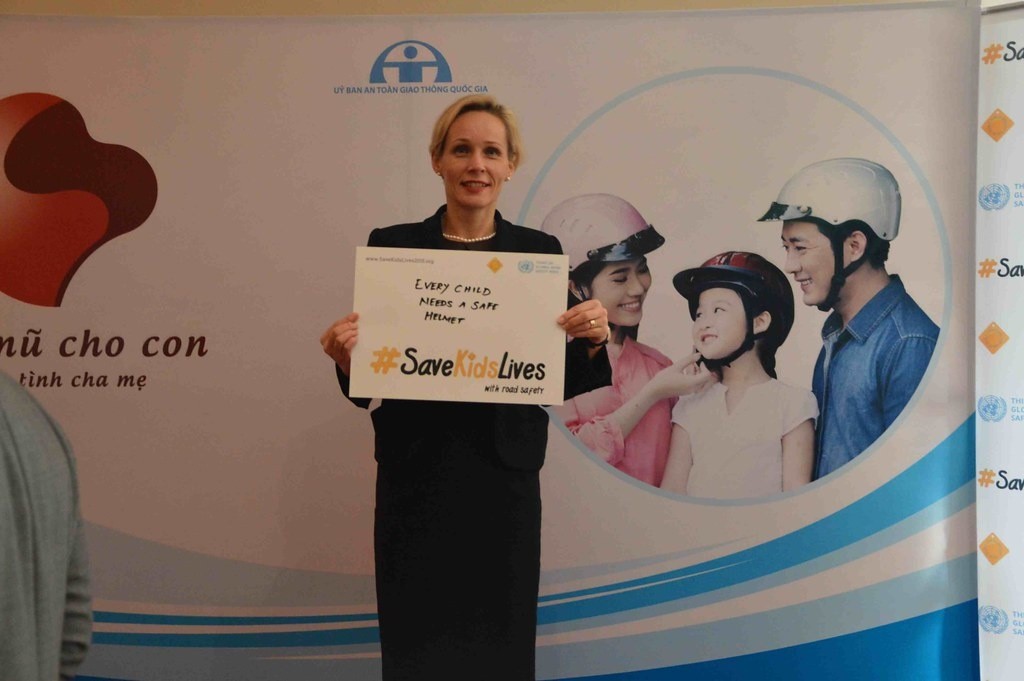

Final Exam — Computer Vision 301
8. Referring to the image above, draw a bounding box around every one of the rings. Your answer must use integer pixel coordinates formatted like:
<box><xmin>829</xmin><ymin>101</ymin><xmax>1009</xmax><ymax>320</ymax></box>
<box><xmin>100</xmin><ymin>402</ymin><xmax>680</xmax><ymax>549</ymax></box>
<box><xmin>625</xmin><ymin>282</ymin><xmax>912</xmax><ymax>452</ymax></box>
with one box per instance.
<box><xmin>590</xmin><ymin>319</ymin><xmax>596</xmax><ymax>327</ymax></box>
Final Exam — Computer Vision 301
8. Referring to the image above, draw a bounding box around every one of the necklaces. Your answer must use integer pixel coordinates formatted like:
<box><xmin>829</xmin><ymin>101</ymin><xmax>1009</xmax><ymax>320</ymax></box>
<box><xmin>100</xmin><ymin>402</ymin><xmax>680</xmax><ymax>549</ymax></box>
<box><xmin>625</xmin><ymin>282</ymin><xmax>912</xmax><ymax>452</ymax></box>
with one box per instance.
<box><xmin>443</xmin><ymin>231</ymin><xmax>496</xmax><ymax>242</ymax></box>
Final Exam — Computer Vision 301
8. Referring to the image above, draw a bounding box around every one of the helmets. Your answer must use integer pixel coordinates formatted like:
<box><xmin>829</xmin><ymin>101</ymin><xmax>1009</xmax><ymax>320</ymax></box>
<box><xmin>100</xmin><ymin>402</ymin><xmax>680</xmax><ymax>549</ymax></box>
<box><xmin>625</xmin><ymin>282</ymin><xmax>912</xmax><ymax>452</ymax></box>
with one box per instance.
<box><xmin>672</xmin><ymin>251</ymin><xmax>795</xmax><ymax>345</ymax></box>
<box><xmin>757</xmin><ymin>158</ymin><xmax>901</xmax><ymax>242</ymax></box>
<box><xmin>540</xmin><ymin>194</ymin><xmax>665</xmax><ymax>287</ymax></box>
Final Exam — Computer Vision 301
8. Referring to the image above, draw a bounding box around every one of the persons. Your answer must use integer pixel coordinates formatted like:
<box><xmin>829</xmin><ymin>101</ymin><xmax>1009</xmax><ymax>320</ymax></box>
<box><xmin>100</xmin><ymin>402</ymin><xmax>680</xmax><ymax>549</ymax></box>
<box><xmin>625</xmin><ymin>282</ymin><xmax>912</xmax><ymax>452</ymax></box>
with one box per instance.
<box><xmin>318</xmin><ymin>93</ymin><xmax>609</xmax><ymax>681</ymax></box>
<box><xmin>540</xmin><ymin>193</ymin><xmax>711</xmax><ymax>488</ymax></box>
<box><xmin>755</xmin><ymin>158</ymin><xmax>940</xmax><ymax>483</ymax></box>
<box><xmin>659</xmin><ymin>252</ymin><xmax>819</xmax><ymax>499</ymax></box>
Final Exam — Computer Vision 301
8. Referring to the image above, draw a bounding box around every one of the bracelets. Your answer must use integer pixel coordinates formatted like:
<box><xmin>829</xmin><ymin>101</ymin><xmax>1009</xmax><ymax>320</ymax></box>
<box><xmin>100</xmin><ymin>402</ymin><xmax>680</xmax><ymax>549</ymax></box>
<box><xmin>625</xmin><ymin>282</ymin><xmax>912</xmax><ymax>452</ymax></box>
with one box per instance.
<box><xmin>587</xmin><ymin>333</ymin><xmax>608</xmax><ymax>346</ymax></box>
